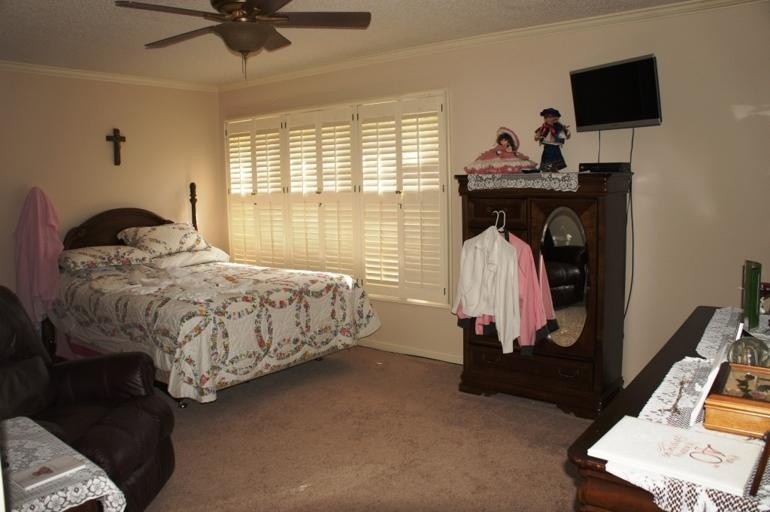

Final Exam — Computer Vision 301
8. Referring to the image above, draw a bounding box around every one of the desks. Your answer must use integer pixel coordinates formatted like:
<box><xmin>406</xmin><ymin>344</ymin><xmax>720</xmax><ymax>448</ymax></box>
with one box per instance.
<box><xmin>567</xmin><ymin>306</ymin><xmax>770</xmax><ymax>512</ymax></box>
<box><xmin>0</xmin><ymin>416</ymin><xmax>127</xmax><ymax>512</ymax></box>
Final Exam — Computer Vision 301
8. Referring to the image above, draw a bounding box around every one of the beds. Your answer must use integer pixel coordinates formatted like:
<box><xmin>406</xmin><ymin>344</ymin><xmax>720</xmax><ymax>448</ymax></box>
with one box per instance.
<box><xmin>34</xmin><ymin>182</ymin><xmax>381</xmax><ymax>408</ymax></box>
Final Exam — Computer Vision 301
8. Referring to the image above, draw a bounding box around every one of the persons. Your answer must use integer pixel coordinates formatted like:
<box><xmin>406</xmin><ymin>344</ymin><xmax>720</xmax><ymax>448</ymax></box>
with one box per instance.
<box><xmin>533</xmin><ymin>107</ymin><xmax>571</xmax><ymax>174</ymax></box>
<box><xmin>464</xmin><ymin>127</ymin><xmax>538</xmax><ymax>174</ymax></box>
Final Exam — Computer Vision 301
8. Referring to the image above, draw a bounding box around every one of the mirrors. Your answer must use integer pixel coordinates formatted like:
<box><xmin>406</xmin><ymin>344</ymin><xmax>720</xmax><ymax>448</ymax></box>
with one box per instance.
<box><xmin>540</xmin><ymin>207</ymin><xmax>589</xmax><ymax>347</ymax></box>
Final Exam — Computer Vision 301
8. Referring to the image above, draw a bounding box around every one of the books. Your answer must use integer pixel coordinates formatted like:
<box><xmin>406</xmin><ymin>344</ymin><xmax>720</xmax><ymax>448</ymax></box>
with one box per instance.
<box><xmin>9</xmin><ymin>455</ymin><xmax>86</xmax><ymax>491</ymax></box>
<box><xmin>586</xmin><ymin>413</ymin><xmax>770</xmax><ymax>497</ymax></box>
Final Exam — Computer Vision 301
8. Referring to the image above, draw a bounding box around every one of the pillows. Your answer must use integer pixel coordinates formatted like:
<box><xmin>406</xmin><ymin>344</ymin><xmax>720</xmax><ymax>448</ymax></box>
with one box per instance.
<box><xmin>58</xmin><ymin>246</ymin><xmax>152</xmax><ymax>272</ymax></box>
<box><xmin>118</xmin><ymin>223</ymin><xmax>210</xmax><ymax>259</ymax></box>
<box><xmin>149</xmin><ymin>247</ymin><xmax>231</xmax><ymax>269</ymax></box>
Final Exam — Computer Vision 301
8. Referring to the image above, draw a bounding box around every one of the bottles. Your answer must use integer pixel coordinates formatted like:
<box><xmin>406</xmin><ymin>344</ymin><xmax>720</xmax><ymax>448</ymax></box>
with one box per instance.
<box><xmin>726</xmin><ymin>337</ymin><xmax>770</xmax><ymax>371</ymax></box>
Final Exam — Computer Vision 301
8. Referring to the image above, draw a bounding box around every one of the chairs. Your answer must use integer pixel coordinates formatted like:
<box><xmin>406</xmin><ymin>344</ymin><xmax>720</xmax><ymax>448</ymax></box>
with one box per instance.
<box><xmin>0</xmin><ymin>285</ymin><xmax>174</xmax><ymax>512</ymax></box>
<box><xmin>545</xmin><ymin>229</ymin><xmax>585</xmax><ymax>308</ymax></box>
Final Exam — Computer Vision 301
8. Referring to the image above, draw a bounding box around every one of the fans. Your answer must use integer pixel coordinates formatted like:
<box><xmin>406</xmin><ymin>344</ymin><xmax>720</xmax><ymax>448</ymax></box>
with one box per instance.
<box><xmin>114</xmin><ymin>0</ymin><xmax>370</xmax><ymax>51</ymax></box>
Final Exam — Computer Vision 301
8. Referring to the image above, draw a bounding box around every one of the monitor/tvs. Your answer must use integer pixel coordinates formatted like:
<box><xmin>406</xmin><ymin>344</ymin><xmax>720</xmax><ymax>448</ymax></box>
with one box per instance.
<box><xmin>570</xmin><ymin>54</ymin><xmax>662</xmax><ymax>132</ymax></box>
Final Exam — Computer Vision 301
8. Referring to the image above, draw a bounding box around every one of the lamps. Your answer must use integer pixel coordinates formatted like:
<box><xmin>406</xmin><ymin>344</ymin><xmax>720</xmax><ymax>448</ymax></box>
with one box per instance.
<box><xmin>215</xmin><ymin>22</ymin><xmax>268</xmax><ymax>79</ymax></box>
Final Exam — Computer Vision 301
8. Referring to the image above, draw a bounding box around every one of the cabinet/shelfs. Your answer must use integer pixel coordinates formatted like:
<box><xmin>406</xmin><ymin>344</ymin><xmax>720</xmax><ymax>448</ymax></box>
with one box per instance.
<box><xmin>455</xmin><ymin>171</ymin><xmax>635</xmax><ymax>420</ymax></box>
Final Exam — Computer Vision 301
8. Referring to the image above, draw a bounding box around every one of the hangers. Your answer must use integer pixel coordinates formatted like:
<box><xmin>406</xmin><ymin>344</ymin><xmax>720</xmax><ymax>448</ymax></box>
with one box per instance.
<box><xmin>492</xmin><ymin>211</ymin><xmax>506</xmax><ymax>232</ymax></box>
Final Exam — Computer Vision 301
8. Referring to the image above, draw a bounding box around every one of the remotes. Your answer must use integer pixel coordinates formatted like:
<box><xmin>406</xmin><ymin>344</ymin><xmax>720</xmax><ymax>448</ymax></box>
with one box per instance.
<box><xmin>521</xmin><ymin>167</ymin><xmax>540</xmax><ymax>173</ymax></box>
